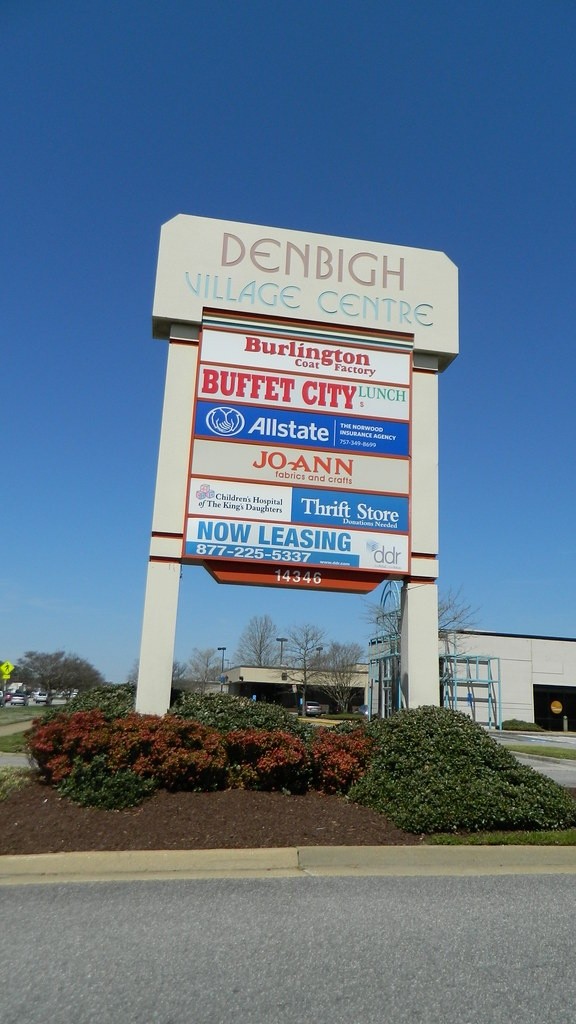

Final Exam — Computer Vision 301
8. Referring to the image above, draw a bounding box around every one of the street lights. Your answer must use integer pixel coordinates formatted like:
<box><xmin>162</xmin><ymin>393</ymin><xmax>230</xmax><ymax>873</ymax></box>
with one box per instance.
<box><xmin>316</xmin><ymin>646</ymin><xmax>323</xmax><ymax>661</ymax></box>
<box><xmin>218</xmin><ymin>647</ymin><xmax>227</xmax><ymax>672</ymax></box>
<box><xmin>276</xmin><ymin>637</ymin><xmax>288</xmax><ymax>665</ymax></box>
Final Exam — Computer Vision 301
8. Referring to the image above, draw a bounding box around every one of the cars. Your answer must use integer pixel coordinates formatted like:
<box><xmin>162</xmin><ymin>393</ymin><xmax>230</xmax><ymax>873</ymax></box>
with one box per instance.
<box><xmin>0</xmin><ymin>687</ymin><xmax>79</xmax><ymax>708</ymax></box>
<box><xmin>297</xmin><ymin>700</ymin><xmax>322</xmax><ymax>718</ymax></box>
